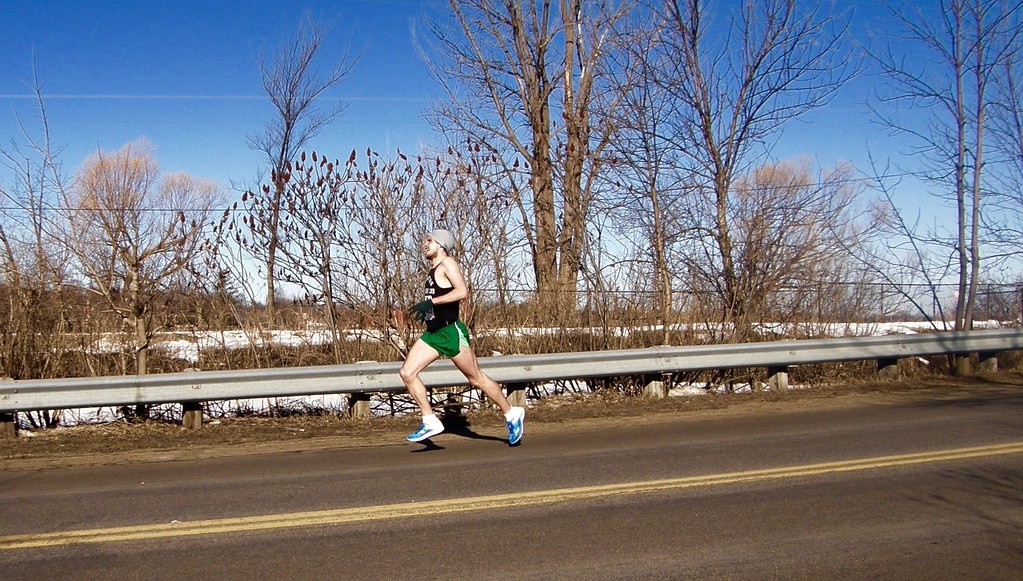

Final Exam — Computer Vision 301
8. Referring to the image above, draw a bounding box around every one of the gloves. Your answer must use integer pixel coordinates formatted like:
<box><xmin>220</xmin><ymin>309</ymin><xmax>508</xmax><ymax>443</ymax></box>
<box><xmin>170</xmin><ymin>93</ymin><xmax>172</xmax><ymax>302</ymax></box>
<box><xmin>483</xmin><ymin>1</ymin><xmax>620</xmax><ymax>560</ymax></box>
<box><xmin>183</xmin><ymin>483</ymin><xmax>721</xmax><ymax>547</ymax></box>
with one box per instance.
<box><xmin>407</xmin><ymin>299</ymin><xmax>434</xmax><ymax>325</ymax></box>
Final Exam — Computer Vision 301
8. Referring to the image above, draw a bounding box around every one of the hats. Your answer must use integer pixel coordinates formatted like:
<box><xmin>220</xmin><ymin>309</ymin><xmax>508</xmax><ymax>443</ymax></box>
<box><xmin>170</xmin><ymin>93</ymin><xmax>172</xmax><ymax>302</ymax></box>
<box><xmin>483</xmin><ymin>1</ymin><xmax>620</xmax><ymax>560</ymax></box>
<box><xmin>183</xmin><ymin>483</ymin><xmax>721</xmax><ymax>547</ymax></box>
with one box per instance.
<box><xmin>428</xmin><ymin>229</ymin><xmax>455</xmax><ymax>254</ymax></box>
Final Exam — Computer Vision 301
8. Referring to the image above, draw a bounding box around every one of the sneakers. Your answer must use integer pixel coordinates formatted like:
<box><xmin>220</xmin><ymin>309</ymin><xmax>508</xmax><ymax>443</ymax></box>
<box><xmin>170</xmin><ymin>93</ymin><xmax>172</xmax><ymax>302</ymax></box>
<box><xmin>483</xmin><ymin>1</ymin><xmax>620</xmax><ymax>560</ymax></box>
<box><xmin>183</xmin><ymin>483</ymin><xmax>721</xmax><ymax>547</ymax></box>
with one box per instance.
<box><xmin>407</xmin><ymin>423</ymin><xmax>445</xmax><ymax>441</ymax></box>
<box><xmin>503</xmin><ymin>407</ymin><xmax>525</xmax><ymax>444</ymax></box>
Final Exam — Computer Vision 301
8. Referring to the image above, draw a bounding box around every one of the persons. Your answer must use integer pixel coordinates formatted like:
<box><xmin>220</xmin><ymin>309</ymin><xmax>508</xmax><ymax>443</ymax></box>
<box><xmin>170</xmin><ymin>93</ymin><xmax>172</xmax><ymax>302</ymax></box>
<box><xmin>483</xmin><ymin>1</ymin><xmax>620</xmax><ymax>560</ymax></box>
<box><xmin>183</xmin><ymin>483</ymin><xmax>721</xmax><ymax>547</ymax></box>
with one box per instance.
<box><xmin>398</xmin><ymin>229</ymin><xmax>525</xmax><ymax>444</ymax></box>
